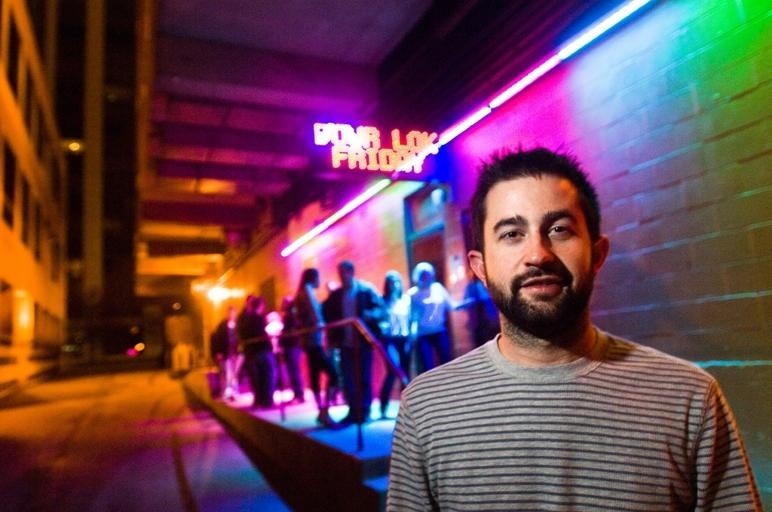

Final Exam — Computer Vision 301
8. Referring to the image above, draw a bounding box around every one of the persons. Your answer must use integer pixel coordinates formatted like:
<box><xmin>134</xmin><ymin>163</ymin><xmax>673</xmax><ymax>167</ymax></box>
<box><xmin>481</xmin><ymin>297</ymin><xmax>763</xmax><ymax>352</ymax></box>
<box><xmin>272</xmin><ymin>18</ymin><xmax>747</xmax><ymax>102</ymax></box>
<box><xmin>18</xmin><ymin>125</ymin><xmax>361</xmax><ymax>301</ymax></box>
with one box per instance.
<box><xmin>209</xmin><ymin>293</ymin><xmax>305</xmax><ymax>408</ymax></box>
<box><xmin>293</xmin><ymin>260</ymin><xmax>453</xmax><ymax>432</ymax></box>
<box><xmin>453</xmin><ymin>278</ymin><xmax>500</xmax><ymax>349</ymax></box>
<box><xmin>385</xmin><ymin>147</ymin><xmax>764</xmax><ymax>512</ymax></box>
